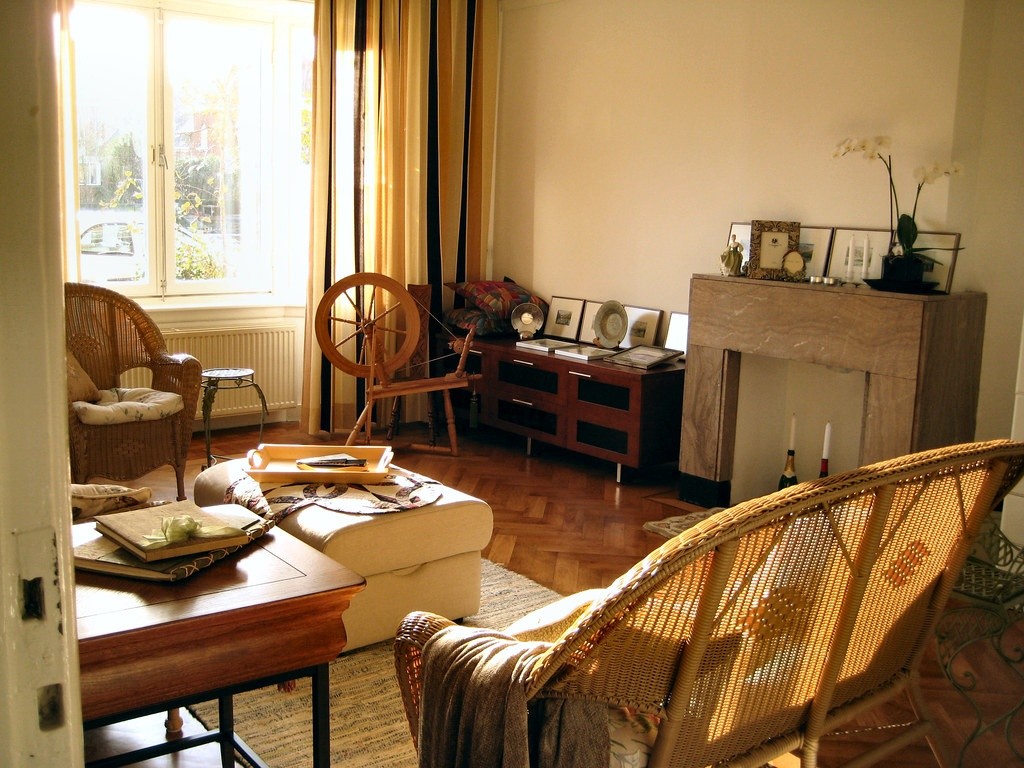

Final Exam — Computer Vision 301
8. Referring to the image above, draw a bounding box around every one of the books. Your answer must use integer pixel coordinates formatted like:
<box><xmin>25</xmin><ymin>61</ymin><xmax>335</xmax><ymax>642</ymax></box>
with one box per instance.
<box><xmin>74</xmin><ymin>500</ymin><xmax>276</xmax><ymax>581</ymax></box>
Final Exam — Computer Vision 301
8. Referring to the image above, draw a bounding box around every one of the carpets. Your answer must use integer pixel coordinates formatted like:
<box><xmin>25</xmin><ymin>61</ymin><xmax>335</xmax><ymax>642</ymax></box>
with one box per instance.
<box><xmin>642</xmin><ymin>507</ymin><xmax>726</xmax><ymax>539</ymax></box>
<box><xmin>190</xmin><ymin>554</ymin><xmax>563</xmax><ymax>768</ymax></box>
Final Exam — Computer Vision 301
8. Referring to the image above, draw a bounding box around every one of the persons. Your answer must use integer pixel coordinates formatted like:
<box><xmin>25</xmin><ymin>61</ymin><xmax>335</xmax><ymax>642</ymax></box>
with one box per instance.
<box><xmin>727</xmin><ymin>235</ymin><xmax>743</xmax><ymax>274</ymax></box>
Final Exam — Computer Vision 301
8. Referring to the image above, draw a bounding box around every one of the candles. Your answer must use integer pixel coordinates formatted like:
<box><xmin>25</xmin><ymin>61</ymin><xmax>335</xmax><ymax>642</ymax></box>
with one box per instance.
<box><xmin>821</xmin><ymin>419</ymin><xmax>831</xmax><ymax>459</ymax></box>
<box><xmin>848</xmin><ymin>233</ymin><xmax>856</xmax><ymax>270</ymax></box>
<box><xmin>863</xmin><ymin>233</ymin><xmax>870</xmax><ymax>273</ymax></box>
<box><xmin>789</xmin><ymin>411</ymin><xmax>797</xmax><ymax>450</ymax></box>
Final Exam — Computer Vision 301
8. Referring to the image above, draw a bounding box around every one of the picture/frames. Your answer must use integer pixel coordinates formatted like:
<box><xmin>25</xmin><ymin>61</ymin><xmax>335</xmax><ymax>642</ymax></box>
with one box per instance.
<box><xmin>603</xmin><ymin>342</ymin><xmax>685</xmax><ymax>370</ymax></box>
<box><xmin>892</xmin><ymin>231</ymin><xmax>962</xmax><ymax>296</ymax></box>
<box><xmin>577</xmin><ymin>300</ymin><xmax>625</xmax><ymax>349</ymax></box>
<box><xmin>662</xmin><ymin>310</ymin><xmax>688</xmax><ymax>359</ymax></box>
<box><xmin>826</xmin><ymin>227</ymin><xmax>894</xmax><ymax>285</ymax></box>
<box><xmin>747</xmin><ymin>219</ymin><xmax>800</xmax><ymax>282</ymax></box>
<box><xmin>799</xmin><ymin>224</ymin><xmax>834</xmax><ymax>282</ymax></box>
<box><xmin>618</xmin><ymin>304</ymin><xmax>665</xmax><ymax>349</ymax></box>
<box><xmin>726</xmin><ymin>221</ymin><xmax>751</xmax><ymax>275</ymax></box>
<box><xmin>542</xmin><ymin>294</ymin><xmax>586</xmax><ymax>343</ymax></box>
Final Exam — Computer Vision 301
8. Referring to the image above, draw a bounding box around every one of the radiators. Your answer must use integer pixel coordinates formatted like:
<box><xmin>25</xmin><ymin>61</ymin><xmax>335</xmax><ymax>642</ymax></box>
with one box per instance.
<box><xmin>120</xmin><ymin>322</ymin><xmax>304</xmax><ymax>420</ymax></box>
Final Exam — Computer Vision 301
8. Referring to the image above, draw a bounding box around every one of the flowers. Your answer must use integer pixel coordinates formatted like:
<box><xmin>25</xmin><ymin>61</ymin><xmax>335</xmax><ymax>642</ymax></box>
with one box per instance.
<box><xmin>831</xmin><ymin>134</ymin><xmax>965</xmax><ymax>267</ymax></box>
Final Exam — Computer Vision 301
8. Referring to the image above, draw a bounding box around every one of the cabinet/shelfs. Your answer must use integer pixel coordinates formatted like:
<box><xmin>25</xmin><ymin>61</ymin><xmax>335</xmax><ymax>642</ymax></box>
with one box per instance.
<box><xmin>449</xmin><ymin>335</ymin><xmax>689</xmax><ymax>486</ymax></box>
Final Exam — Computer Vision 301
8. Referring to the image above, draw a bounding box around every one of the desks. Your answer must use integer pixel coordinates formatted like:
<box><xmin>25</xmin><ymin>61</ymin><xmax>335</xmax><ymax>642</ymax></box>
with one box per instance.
<box><xmin>932</xmin><ymin>514</ymin><xmax>1024</xmax><ymax>768</ymax></box>
<box><xmin>72</xmin><ymin>503</ymin><xmax>368</xmax><ymax>768</ymax></box>
<box><xmin>200</xmin><ymin>367</ymin><xmax>270</xmax><ymax>472</ymax></box>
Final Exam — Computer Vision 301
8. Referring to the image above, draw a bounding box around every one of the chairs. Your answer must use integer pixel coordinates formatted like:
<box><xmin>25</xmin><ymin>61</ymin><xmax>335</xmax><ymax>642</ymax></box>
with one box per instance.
<box><xmin>65</xmin><ymin>281</ymin><xmax>216</xmax><ymax>499</ymax></box>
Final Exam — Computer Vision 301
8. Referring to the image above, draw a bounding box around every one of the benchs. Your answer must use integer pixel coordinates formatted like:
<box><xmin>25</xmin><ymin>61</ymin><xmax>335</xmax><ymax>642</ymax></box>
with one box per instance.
<box><xmin>193</xmin><ymin>458</ymin><xmax>494</xmax><ymax>647</ymax></box>
<box><xmin>393</xmin><ymin>438</ymin><xmax>1024</xmax><ymax>768</ymax></box>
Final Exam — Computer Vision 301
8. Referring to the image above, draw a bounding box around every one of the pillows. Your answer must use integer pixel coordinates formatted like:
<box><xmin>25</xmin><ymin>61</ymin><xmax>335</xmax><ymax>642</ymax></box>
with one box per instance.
<box><xmin>71</xmin><ymin>482</ymin><xmax>153</xmax><ymax>520</ymax></box>
<box><xmin>66</xmin><ymin>349</ymin><xmax>102</xmax><ymax>401</ymax></box>
<box><xmin>442</xmin><ymin>274</ymin><xmax>550</xmax><ymax>321</ymax></box>
<box><xmin>442</xmin><ymin>307</ymin><xmax>520</xmax><ymax>339</ymax></box>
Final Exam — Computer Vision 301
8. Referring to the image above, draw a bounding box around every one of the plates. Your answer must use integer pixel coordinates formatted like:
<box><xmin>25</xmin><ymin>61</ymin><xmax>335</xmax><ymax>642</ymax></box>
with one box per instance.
<box><xmin>511</xmin><ymin>303</ymin><xmax>544</xmax><ymax>334</ymax></box>
<box><xmin>594</xmin><ymin>300</ymin><xmax>628</xmax><ymax>349</ymax></box>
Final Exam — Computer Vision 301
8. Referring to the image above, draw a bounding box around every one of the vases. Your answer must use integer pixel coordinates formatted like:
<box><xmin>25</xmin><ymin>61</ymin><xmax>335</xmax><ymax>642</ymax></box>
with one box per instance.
<box><xmin>863</xmin><ymin>255</ymin><xmax>940</xmax><ymax>294</ymax></box>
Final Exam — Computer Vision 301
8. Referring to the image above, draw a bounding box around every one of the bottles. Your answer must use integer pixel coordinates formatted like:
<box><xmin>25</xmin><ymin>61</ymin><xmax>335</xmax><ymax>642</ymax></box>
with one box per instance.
<box><xmin>777</xmin><ymin>449</ymin><xmax>798</xmax><ymax>491</ymax></box>
<box><xmin>819</xmin><ymin>458</ymin><xmax>829</xmax><ymax>478</ymax></box>
